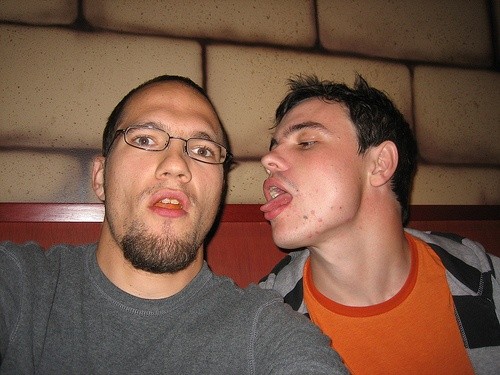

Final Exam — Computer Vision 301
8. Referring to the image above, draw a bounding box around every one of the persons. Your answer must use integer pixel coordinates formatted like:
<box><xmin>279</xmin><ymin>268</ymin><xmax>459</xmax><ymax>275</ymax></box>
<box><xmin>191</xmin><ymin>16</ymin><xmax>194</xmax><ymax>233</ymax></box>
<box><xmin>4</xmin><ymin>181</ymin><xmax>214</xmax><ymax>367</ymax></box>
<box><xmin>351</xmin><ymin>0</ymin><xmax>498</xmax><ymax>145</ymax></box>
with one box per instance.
<box><xmin>257</xmin><ymin>71</ymin><xmax>500</xmax><ymax>374</ymax></box>
<box><xmin>1</xmin><ymin>74</ymin><xmax>350</xmax><ymax>374</ymax></box>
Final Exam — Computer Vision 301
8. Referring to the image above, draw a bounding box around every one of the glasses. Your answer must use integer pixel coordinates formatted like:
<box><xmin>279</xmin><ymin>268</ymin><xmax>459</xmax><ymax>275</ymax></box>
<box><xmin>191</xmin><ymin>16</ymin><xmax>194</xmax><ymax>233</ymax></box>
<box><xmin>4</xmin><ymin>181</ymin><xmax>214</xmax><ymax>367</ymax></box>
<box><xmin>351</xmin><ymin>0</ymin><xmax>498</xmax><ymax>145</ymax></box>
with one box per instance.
<box><xmin>108</xmin><ymin>124</ymin><xmax>234</xmax><ymax>165</ymax></box>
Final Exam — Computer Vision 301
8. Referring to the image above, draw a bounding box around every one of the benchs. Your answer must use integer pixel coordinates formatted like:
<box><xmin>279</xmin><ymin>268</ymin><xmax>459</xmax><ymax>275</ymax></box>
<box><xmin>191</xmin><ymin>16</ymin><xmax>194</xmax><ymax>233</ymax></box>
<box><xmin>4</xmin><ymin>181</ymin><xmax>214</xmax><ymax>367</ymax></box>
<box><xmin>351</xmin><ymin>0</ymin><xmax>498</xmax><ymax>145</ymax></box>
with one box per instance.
<box><xmin>0</xmin><ymin>203</ymin><xmax>500</xmax><ymax>290</ymax></box>
